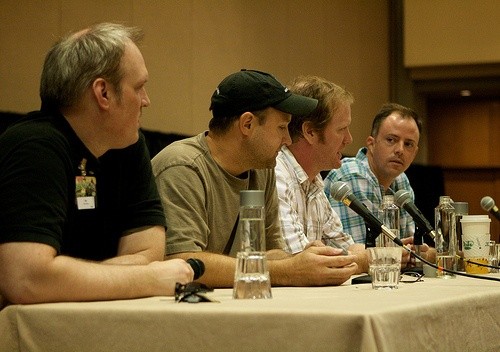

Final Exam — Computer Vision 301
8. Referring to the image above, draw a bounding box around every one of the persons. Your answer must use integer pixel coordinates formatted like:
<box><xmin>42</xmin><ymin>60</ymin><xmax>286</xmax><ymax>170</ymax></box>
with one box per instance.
<box><xmin>0</xmin><ymin>22</ymin><xmax>194</xmax><ymax>311</ymax></box>
<box><xmin>274</xmin><ymin>75</ymin><xmax>429</xmax><ymax>275</ymax></box>
<box><xmin>325</xmin><ymin>103</ymin><xmax>464</xmax><ymax>269</ymax></box>
<box><xmin>151</xmin><ymin>68</ymin><xmax>358</xmax><ymax>287</ymax></box>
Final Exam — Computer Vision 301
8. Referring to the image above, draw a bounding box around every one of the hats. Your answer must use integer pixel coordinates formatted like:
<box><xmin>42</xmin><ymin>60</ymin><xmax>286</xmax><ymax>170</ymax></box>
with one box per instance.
<box><xmin>212</xmin><ymin>68</ymin><xmax>319</xmax><ymax>116</ymax></box>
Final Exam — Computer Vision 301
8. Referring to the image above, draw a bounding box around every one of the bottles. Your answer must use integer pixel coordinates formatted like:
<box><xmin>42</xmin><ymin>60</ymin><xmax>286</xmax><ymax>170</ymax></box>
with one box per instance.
<box><xmin>434</xmin><ymin>196</ymin><xmax>457</xmax><ymax>280</ymax></box>
<box><xmin>378</xmin><ymin>194</ymin><xmax>400</xmax><ymax>247</ymax></box>
<box><xmin>239</xmin><ymin>190</ymin><xmax>267</xmax><ymax>254</ymax></box>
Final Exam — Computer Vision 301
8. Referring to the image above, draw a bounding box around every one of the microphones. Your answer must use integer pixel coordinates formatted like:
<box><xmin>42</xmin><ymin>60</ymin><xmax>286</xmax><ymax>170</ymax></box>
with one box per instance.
<box><xmin>393</xmin><ymin>189</ymin><xmax>437</xmax><ymax>241</ymax></box>
<box><xmin>329</xmin><ymin>181</ymin><xmax>404</xmax><ymax>246</ymax></box>
<box><xmin>480</xmin><ymin>195</ymin><xmax>500</xmax><ymax>222</ymax></box>
<box><xmin>185</xmin><ymin>257</ymin><xmax>206</xmax><ymax>280</ymax></box>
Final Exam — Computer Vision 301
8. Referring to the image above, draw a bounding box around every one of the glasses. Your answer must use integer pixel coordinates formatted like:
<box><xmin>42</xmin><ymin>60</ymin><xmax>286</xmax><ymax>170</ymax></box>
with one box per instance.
<box><xmin>400</xmin><ymin>271</ymin><xmax>425</xmax><ymax>283</ymax></box>
<box><xmin>174</xmin><ymin>281</ymin><xmax>215</xmax><ymax>303</ymax></box>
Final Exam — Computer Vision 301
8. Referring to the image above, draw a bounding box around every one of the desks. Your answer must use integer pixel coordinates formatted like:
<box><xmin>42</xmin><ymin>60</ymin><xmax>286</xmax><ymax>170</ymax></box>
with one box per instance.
<box><xmin>0</xmin><ymin>268</ymin><xmax>500</xmax><ymax>352</ymax></box>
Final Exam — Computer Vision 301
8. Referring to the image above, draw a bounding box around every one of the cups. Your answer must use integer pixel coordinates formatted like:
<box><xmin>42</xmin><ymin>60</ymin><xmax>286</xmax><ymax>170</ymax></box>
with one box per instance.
<box><xmin>489</xmin><ymin>244</ymin><xmax>500</xmax><ymax>273</ymax></box>
<box><xmin>460</xmin><ymin>214</ymin><xmax>492</xmax><ymax>275</ymax></box>
<box><xmin>365</xmin><ymin>245</ymin><xmax>402</xmax><ymax>290</ymax></box>
<box><xmin>232</xmin><ymin>250</ymin><xmax>273</xmax><ymax>299</ymax></box>
